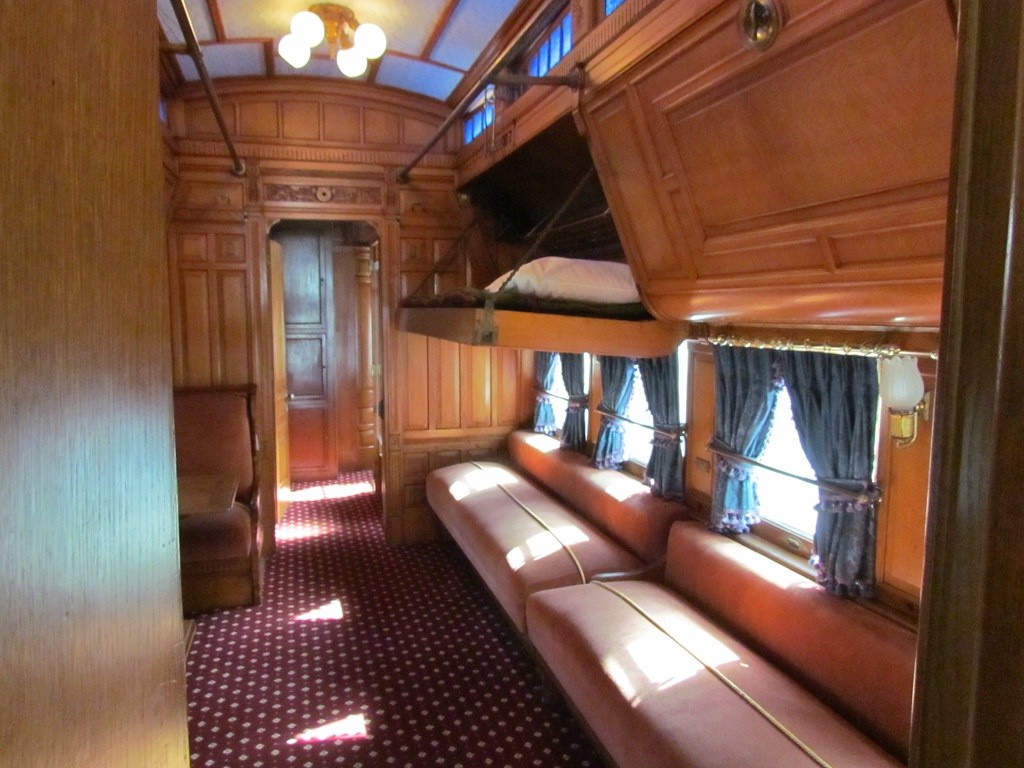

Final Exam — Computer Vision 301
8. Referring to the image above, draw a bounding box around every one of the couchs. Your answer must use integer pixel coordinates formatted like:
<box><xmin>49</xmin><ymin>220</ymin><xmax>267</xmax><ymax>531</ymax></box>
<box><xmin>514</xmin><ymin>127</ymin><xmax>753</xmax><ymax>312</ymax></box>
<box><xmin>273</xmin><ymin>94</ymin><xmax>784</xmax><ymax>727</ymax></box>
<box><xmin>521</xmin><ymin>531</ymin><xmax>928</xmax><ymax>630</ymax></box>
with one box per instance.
<box><xmin>173</xmin><ymin>383</ymin><xmax>266</xmax><ymax>611</ymax></box>
<box><xmin>426</xmin><ymin>431</ymin><xmax>685</xmax><ymax>634</ymax></box>
<box><xmin>525</xmin><ymin>521</ymin><xmax>919</xmax><ymax>768</ymax></box>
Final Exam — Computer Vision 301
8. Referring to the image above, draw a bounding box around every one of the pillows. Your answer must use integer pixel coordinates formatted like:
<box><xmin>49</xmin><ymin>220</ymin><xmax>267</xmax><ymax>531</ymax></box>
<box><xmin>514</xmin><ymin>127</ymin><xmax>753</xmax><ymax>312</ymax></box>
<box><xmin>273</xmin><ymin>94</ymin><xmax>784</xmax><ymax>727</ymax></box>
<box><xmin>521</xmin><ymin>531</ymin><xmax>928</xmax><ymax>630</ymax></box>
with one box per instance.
<box><xmin>484</xmin><ymin>256</ymin><xmax>639</xmax><ymax>304</ymax></box>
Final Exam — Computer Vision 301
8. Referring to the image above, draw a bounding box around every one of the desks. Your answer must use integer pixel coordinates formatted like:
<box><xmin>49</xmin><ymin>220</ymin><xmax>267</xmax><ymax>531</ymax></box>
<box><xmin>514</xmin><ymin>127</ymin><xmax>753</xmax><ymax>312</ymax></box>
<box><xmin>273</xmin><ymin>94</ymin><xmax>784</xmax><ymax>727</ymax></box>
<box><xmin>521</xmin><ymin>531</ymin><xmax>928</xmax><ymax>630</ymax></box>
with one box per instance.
<box><xmin>178</xmin><ymin>472</ymin><xmax>240</xmax><ymax>517</ymax></box>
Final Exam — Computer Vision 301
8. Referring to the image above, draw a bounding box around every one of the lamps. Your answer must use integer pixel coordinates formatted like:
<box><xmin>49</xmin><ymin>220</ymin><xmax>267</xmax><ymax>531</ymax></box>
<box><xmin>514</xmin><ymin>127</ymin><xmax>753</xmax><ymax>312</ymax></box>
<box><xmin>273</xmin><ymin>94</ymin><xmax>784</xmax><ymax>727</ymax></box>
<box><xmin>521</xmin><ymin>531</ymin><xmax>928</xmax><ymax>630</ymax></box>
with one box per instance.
<box><xmin>278</xmin><ymin>3</ymin><xmax>386</xmax><ymax>78</ymax></box>
<box><xmin>877</xmin><ymin>356</ymin><xmax>932</xmax><ymax>449</ymax></box>
